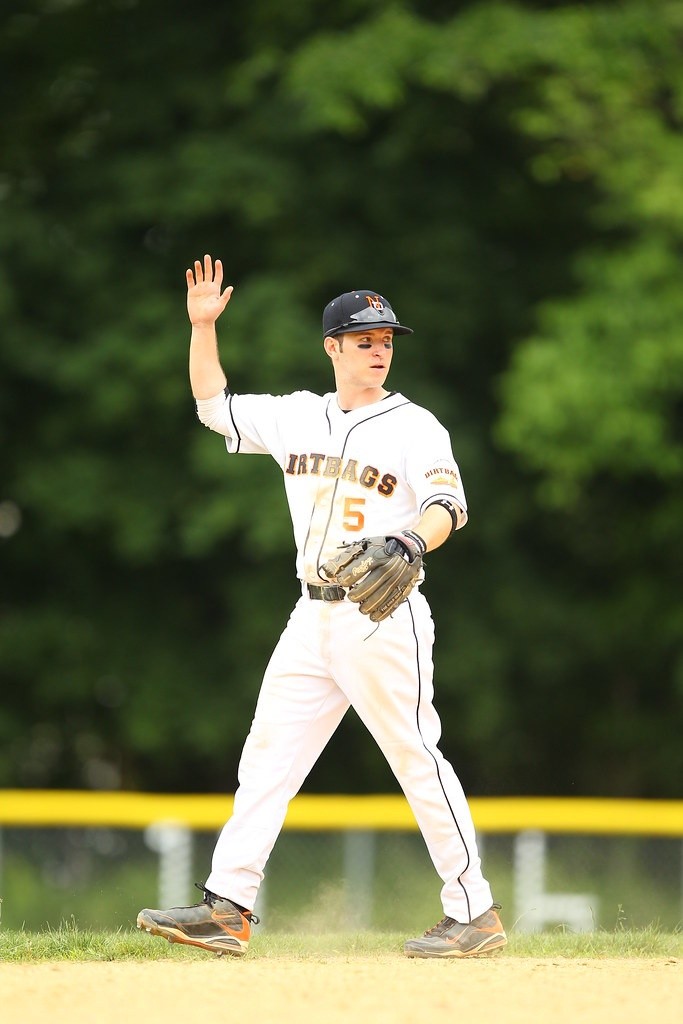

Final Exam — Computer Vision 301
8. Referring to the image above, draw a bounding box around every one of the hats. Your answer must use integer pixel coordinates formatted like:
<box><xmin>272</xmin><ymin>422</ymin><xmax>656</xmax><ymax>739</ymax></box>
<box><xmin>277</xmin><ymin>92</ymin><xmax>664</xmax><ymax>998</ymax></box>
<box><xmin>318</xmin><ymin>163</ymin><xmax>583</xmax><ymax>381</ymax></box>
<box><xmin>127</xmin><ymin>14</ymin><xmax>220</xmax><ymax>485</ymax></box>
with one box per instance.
<box><xmin>322</xmin><ymin>290</ymin><xmax>413</xmax><ymax>341</ymax></box>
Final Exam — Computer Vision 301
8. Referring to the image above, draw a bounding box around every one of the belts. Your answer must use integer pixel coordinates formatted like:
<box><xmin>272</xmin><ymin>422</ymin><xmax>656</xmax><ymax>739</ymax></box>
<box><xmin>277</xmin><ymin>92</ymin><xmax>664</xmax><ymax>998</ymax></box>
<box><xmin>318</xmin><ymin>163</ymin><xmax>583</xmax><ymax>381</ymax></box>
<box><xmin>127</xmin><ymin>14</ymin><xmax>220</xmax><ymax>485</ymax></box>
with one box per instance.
<box><xmin>300</xmin><ymin>580</ymin><xmax>349</xmax><ymax>603</ymax></box>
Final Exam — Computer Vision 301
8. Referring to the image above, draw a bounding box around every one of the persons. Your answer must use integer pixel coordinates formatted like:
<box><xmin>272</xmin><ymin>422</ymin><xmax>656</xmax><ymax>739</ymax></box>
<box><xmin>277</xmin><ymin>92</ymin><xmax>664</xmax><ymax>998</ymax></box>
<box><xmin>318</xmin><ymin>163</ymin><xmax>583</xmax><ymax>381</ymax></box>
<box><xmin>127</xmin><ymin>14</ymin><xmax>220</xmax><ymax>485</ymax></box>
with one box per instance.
<box><xmin>137</xmin><ymin>256</ymin><xmax>510</xmax><ymax>957</ymax></box>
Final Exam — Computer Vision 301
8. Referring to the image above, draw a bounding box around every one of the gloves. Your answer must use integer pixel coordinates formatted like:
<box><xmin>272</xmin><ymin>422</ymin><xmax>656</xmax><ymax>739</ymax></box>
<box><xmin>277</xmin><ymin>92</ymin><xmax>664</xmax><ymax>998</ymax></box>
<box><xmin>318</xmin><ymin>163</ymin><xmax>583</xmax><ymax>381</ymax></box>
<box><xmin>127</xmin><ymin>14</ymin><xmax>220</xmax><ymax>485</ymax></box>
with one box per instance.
<box><xmin>385</xmin><ymin>528</ymin><xmax>426</xmax><ymax>555</ymax></box>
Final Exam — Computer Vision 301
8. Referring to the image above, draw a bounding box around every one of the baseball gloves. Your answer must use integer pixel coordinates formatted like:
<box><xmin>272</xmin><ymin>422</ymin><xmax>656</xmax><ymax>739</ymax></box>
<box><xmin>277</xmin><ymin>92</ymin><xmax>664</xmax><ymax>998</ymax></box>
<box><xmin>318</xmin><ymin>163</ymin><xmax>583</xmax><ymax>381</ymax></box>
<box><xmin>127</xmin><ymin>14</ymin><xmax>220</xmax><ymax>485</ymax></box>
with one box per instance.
<box><xmin>317</xmin><ymin>524</ymin><xmax>431</xmax><ymax>626</ymax></box>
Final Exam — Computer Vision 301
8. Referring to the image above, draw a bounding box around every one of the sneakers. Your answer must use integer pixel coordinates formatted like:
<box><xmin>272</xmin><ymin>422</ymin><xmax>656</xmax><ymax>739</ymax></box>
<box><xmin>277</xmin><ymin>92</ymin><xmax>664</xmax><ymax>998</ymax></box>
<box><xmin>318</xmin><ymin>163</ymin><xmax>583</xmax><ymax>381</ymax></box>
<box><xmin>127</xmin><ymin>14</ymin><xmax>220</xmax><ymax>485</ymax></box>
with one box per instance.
<box><xmin>403</xmin><ymin>904</ymin><xmax>507</xmax><ymax>958</ymax></box>
<box><xmin>136</xmin><ymin>882</ymin><xmax>260</xmax><ymax>957</ymax></box>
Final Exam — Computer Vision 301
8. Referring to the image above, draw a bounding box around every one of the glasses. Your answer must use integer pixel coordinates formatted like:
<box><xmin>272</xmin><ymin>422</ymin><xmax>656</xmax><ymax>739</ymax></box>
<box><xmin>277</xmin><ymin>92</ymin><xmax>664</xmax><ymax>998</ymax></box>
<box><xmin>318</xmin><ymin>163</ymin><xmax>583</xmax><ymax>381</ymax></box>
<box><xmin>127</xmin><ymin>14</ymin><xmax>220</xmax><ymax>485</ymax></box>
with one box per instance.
<box><xmin>324</xmin><ymin>306</ymin><xmax>400</xmax><ymax>337</ymax></box>
